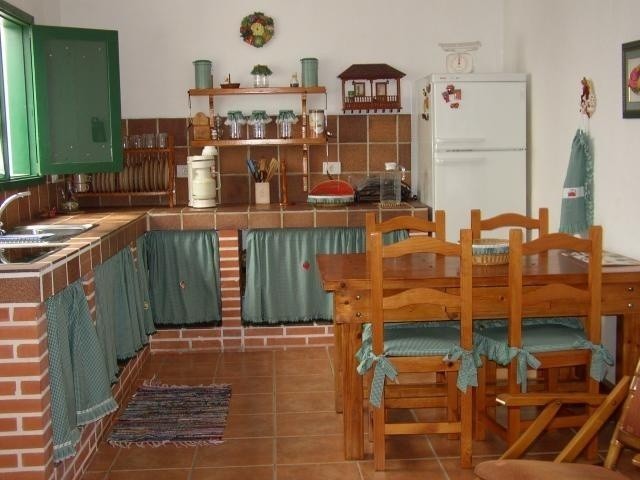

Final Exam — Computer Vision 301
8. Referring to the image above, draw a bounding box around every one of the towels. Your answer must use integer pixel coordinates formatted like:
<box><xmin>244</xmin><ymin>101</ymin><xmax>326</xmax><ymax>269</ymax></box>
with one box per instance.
<box><xmin>558</xmin><ymin>128</ymin><xmax>594</xmax><ymax>239</ymax></box>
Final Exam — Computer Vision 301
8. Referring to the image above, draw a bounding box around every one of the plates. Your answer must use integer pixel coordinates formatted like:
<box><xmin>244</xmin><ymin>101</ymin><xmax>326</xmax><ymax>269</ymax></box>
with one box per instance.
<box><xmin>92</xmin><ymin>152</ymin><xmax>171</xmax><ymax>191</ymax></box>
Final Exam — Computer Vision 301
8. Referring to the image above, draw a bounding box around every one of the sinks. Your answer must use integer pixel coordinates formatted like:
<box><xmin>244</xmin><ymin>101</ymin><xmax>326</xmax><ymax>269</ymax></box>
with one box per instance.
<box><xmin>1</xmin><ymin>223</ymin><xmax>100</xmax><ymax>242</ymax></box>
<box><xmin>0</xmin><ymin>239</ymin><xmax>70</xmax><ymax>264</ymax></box>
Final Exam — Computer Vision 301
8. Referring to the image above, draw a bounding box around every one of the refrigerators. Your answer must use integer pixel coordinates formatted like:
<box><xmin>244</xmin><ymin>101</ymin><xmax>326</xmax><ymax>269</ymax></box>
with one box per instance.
<box><xmin>414</xmin><ymin>73</ymin><xmax>527</xmax><ymax>248</ymax></box>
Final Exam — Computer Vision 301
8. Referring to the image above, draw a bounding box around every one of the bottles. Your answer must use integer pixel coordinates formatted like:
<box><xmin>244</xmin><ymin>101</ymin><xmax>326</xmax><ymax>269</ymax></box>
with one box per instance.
<box><xmin>380</xmin><ymin>161</ymin><xmax>401</xmax><ymax>207</ymax></box>
<box><xmin>224</xmin><ymin>65</ymin><xmax>325</xmax><ymax>140</ymax></box>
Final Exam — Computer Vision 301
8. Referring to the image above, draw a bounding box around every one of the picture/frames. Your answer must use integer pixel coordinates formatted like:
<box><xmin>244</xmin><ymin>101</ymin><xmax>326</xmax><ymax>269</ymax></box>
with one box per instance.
<box><xmin>621</xmin><ymin>39</ymin><xmax>640</xmax><ymax>120</ymax></box>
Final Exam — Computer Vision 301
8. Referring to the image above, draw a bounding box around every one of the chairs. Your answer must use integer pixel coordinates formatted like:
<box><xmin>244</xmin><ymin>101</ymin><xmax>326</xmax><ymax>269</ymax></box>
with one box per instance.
<box><xmin>473</xmin><ymin>226</ymin><xmax>607</xmax><ymax>463</ymax></box>
<box><xmin>363</xmin><ymin>210</ymin><xmax>447</xmax><ymax>386</ymax></box>
<box><xmin>474</xmin><ymin>362</ymin><xmax>640</xmax><ymax>480</ymax></box>
<box><xmin>366</xmin><ymin>228</ymin><xmax>478</xmax><ymax>471</ymax></box>
<box><xmin>469</xmin><ymin>209</ymin><xmax>578</xmax><ymax>379</ymax></box>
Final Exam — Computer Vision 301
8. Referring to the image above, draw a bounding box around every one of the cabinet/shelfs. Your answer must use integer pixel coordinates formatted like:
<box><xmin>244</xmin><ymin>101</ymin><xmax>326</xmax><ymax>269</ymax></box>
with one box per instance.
<box><xmin>188</xmin><ymin>87</ymin><xmax>326</xmax><ymax>192</ymax></box>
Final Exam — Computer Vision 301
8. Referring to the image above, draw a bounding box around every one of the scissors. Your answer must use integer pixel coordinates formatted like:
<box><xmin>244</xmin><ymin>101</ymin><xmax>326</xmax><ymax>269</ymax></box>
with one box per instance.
<box><xmin>253</xmin><ymin>170</ymin><xmax>266</xmax><ymax>183</ymax></box>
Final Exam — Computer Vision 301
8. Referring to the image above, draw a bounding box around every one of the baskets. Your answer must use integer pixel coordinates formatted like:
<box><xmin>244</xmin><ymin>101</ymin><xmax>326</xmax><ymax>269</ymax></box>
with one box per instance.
<box><xmin>457</xmin><ymin>238</ymin><xmax>509</xmax><ymax>265</ymax></box>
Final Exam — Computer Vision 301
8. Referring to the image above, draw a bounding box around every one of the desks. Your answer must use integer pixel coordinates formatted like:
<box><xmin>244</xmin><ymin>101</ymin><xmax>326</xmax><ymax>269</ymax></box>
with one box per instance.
<box><xmin>317</xmin><ymin>243</ymin><xmax>640</xmax><ymax>461</ymax></box>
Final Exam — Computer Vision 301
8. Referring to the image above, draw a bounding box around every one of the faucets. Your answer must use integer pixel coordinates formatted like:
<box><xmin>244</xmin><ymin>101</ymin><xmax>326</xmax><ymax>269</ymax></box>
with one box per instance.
<box><xmin>0</xmin><ymin>190</ymin><xmax>32</xmax><ymax>216</ymax></box>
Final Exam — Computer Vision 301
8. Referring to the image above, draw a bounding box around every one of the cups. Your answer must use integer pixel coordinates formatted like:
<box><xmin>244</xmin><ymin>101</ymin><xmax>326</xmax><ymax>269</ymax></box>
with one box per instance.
<box><xmin>122</xmin><ymin>133</ymin><xmax>168</xmax><ymax>148</ymax></box>
<box><xmin>73</xmin><ymin>174</ymin><xmax>91</xmax><ymax>193</ymax></box>
<box><xmin>254</xmin><ymin>183</ymin><xmax>270</xmax><ymax>205</ymax></box>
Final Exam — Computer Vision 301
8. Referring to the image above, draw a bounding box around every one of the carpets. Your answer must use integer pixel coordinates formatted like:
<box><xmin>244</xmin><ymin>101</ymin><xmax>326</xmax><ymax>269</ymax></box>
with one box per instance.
<box><xmin>105</xmin><ymin>375</ymin><xmax>233</xmax><ymax>449</ymax></box>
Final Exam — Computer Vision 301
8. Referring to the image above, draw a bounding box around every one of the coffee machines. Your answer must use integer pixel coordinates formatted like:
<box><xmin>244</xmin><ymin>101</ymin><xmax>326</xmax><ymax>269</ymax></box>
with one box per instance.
<box><xmin>187</xmin><ymin>154</ymin><xmax>222</xmax><ymax>209</ymax></box>
